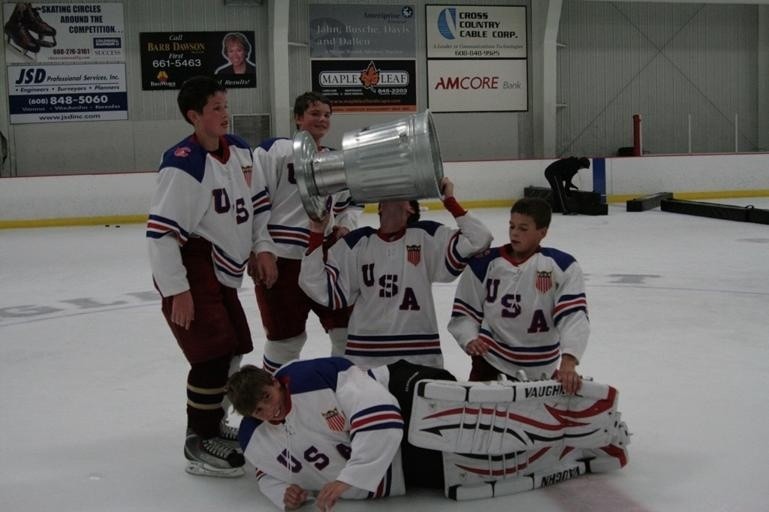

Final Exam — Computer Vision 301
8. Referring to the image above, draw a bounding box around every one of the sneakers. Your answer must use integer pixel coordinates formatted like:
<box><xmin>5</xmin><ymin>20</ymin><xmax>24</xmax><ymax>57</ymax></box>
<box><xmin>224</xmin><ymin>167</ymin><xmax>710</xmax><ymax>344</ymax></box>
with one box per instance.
<box><xmin>184</xmin><ymin>426</ymin><xmax>244</xmax><ymax>469</ymax></box>
<box><xmin>4</xmin><ymin>5</ymin><xmax>55</xmax><ymax>51</ymax></box>
<box><xmin>214</xmin><ymin>421</ymin><xmax>241</xmax><ymax>448</ymax></box>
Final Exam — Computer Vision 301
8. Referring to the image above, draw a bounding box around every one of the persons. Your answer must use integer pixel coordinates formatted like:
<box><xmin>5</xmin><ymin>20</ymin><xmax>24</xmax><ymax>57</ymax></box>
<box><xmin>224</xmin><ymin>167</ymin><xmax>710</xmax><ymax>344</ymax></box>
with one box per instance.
<box><xmin>224</xmin><ymin>359</ymin><xmax>630</xmax><ymax>512</ymax></box>
<box><xmin>446</xmin><ymin>198</ymin><xmax>590</xmax><ymax>394</ymax></box>
<box><xmin>249</xmin><ymin>91</ymin><xmax>354</xmax><ymax>380</ymax></box>
<box><xmin>146</xmin><ymin>74</ymin><xmax>279</xmax><ymax>468</ymax></box>
<box><xmin>214</xmin><ymin>32</ymin><xmax>256</xmax><ymax>77</ymax></box>
<box><xmin>298</xmin><ymin>178</ymin><xmax>495</xmax><ymax>372</ymax></box>
<box><xmin>543</xmin><ymin>156</ymin><xmax>590</xmax><ymax>216</ymax></box>
